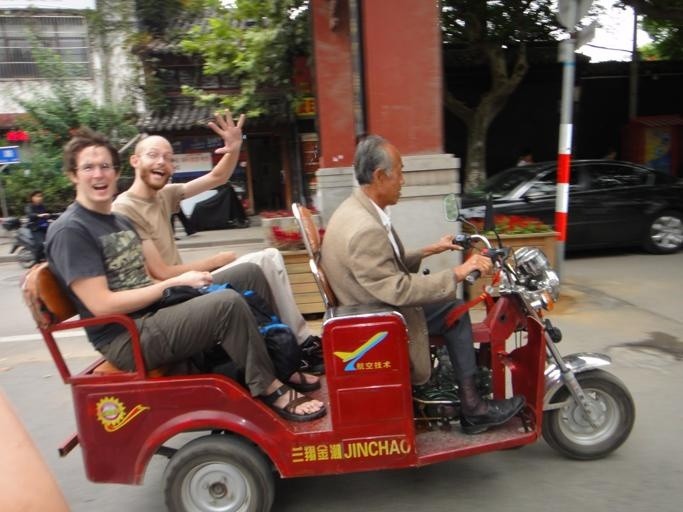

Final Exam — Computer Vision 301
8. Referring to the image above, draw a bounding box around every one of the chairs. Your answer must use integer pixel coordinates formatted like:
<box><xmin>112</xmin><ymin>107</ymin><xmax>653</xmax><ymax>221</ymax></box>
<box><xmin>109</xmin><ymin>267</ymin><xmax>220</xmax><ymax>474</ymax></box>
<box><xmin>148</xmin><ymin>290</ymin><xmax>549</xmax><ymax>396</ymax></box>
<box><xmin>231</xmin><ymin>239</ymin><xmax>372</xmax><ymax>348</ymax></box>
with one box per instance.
<box><xmin>17</xmin><ymin>260</ymin><xmax>147</xmax><ymax>384</ymax></box>
<box><xmin>288</xmin><ymin>202</ymin><xmax>341</xmax><ymax>309</ymax></box>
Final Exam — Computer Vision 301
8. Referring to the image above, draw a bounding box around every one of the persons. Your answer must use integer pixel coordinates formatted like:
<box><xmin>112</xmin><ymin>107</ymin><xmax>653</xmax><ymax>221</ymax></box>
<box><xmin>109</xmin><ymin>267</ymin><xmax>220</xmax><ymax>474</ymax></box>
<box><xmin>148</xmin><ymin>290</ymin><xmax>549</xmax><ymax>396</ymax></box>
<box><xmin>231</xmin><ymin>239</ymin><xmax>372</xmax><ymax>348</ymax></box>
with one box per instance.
<box><xmin>319</xmin><ymin>131</ymin><xmax>527</xmax><ymax>435</ymax></box>
<box><xmin>46</xmin><ymin>123</ymin><xmax>326</xmax><ymax>422</ymax></box>
<box><xmin>109</xmin><ymin>107</ymin><xmax>324</xmax><ymax>375</ymax></box>
<box><xmin>515</xmin><ymin>148</ymin><xmax>534</xmax><ymax>166</ymax></box>
<box><xmin>25</xmin><ymin>191</ymin><xmax>52</xmax><ymax>262</ymax></box>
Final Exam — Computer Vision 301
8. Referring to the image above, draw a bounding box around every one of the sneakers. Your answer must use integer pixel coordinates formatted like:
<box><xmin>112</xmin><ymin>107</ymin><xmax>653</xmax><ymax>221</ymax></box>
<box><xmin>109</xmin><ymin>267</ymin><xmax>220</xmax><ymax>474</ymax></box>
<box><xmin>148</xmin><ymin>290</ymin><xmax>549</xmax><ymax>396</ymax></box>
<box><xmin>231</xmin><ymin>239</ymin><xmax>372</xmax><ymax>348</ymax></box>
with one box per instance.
<box><xmin>300</xmin><ymin>334</ymin><xmax>325</xmax><ymax>374</ymax></box>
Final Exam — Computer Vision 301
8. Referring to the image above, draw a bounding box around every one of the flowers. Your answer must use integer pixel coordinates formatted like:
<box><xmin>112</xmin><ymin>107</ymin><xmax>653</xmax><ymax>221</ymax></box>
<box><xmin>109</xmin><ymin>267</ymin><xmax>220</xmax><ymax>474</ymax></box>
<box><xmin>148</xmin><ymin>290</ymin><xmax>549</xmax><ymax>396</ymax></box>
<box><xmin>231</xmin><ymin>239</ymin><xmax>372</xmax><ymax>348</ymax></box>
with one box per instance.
<box><xmin>465</xmin><ymin>212</ymin><xmax>553</xmax><ymax>236</ymax></box>
<box><xmin>260</xmin><ymin>207</ymin><xmax>325</xmax><ymax>251</ymax></box>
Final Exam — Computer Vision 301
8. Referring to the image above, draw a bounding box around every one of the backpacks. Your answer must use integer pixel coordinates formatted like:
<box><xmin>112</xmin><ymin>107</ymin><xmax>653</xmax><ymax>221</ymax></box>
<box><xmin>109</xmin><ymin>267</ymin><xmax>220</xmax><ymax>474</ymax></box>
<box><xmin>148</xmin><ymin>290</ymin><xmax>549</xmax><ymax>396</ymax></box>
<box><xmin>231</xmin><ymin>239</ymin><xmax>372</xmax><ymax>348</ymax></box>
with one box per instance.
<box><xmin>160</xmin><ymin>280</ymin><xmax>299</xmax><ymax>388</ymax></box>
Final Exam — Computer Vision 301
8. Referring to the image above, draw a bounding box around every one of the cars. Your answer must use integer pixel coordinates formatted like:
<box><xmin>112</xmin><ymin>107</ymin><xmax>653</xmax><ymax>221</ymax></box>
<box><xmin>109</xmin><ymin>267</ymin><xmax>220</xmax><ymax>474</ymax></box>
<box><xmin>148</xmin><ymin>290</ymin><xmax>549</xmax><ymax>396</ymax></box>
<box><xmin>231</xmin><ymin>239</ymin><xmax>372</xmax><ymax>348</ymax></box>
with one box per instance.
<box><xmin>458</xmin><ymin>158</ymin><xmax>683</xmax><ymax>255</ymax></box>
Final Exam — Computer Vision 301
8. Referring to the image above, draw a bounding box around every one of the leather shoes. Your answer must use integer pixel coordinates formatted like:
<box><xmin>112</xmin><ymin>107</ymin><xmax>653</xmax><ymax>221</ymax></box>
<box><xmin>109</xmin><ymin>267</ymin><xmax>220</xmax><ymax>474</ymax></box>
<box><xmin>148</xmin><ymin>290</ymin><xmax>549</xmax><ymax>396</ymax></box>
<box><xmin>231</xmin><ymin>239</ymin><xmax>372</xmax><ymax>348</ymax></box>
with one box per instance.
<box><xmin>461</xmin><ymin>396</ymin><xmax>523</xmax><ymax>434</ymax></box>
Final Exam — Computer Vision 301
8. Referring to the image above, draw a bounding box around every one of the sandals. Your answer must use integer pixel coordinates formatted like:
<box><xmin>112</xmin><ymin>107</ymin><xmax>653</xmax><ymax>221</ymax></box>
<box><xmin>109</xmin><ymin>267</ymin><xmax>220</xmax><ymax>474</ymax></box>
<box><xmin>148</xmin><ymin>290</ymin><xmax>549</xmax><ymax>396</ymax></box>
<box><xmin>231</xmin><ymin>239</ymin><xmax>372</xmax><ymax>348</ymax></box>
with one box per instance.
<box><xmin>285</xmin><ymin>372</ymin><xmax>321</xmax><ymax>392</ymax></box>
<box><xmin>259</xmin><ymin>379</ymin><xmax>326</xmax><ymax>422</ymax></box>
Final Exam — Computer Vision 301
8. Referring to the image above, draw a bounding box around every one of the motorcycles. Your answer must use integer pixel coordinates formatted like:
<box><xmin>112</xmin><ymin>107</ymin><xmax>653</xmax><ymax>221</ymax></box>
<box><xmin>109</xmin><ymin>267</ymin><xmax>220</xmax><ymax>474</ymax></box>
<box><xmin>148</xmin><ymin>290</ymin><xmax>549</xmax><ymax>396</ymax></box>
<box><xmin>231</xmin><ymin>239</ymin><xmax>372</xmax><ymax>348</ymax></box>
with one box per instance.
<box><xmin>22</xmin><ymin>192</ymin><xmax>635</xmax><ymax>512</ymax></box>
<box><xmin>0</xmin><ymin>213</ymin><xmax>61</xmax><ymax>268</ymax></box>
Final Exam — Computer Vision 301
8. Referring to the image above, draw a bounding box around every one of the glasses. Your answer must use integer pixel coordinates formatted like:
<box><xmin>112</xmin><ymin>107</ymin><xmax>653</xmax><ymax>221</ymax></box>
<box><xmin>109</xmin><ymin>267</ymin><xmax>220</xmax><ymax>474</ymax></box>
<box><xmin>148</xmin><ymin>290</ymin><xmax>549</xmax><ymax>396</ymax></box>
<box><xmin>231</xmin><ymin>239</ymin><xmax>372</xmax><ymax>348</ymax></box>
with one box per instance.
<box><xmin>71</xmin><ymin>162</ymin><xmax>120</xmax><ymax>175</ymax></box>
